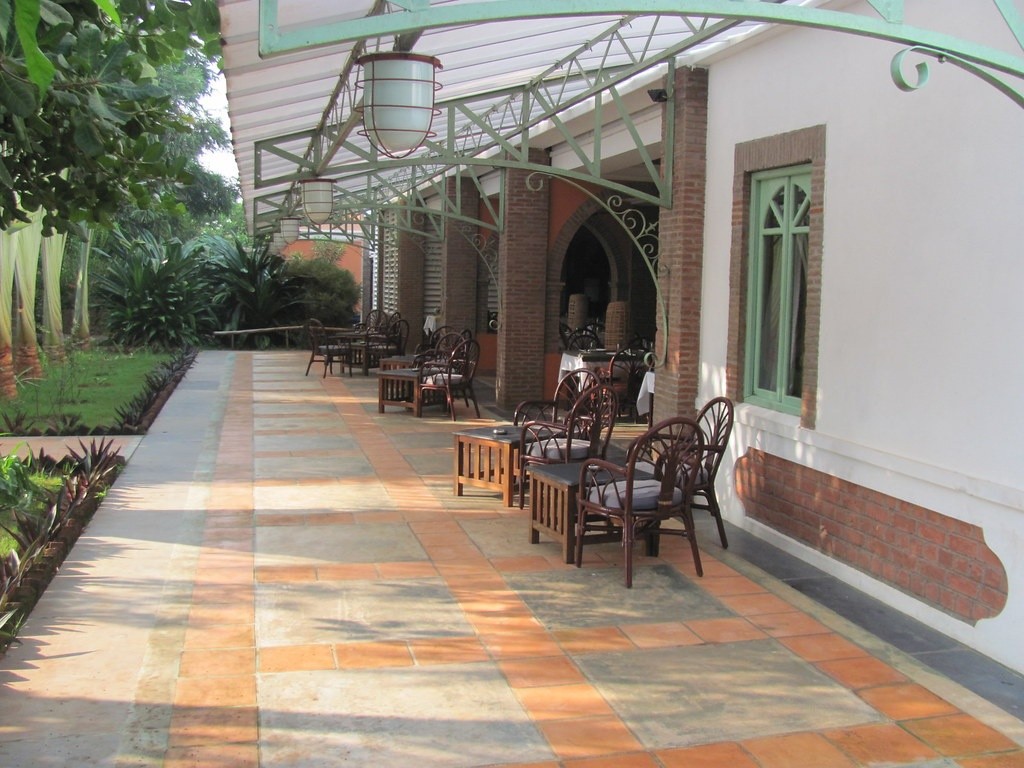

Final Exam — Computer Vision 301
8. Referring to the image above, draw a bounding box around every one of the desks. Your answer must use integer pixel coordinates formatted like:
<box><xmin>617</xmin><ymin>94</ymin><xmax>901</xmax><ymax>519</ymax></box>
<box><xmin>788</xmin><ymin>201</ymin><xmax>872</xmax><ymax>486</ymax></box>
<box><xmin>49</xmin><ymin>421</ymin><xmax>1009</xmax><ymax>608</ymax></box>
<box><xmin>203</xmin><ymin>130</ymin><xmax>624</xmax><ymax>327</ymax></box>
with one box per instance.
<box><xmin>452</xmin><ymin>425</ymin><xmax>564</xmax><ymax>508</ymax></box>
<box><xmin>524</xmin><ymin>462</ymin><xmax>655</xmax><ymax>565</ymax></box>
<box><xmin>336</xmin><ymin>331</ymin><xmax>380</xmax><ymax>376</ymax></box>
<box><xmin>379</xmin><ymin>355</ymin><xmax>430</xmax><ymax>369</ymax></box>
<box><xmin>557</xmin><ymin>350</ymin><xmax>645</xmax><ymax>392</ymax></box>
<box><xmin>378</xmin><ymin>368</ymin><xmax>446</xmax><ymax>416</ymax></box>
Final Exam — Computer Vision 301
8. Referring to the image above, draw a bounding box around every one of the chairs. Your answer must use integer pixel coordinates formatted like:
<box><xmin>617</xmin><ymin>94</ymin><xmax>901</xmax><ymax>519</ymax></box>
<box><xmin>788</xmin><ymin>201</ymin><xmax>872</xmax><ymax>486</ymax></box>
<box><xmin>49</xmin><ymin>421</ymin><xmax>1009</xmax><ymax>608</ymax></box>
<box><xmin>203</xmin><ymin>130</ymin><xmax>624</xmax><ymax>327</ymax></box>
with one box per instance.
<box><xmin>513</xmin><ymin>368</ymin><xmax>603</xmax><ymax>427</ymax></box>
<box><xmin>353</xmin><ymin>310</ymin><xmax>409</xmax><ymax>375</ymax></box>
<box><xmin>520</xmin><ymin>385</ymin><xmax>619</xmax><ymax>510</ymax></box>
<box><xmin>625</xmin><ymin>397</ymin><xmax>734</xmax><ymax>548</ymax></box>
<box><xmin>412</xmin><ymin>326</ymin><xmax>480</xmax><ymax>420</ymax></box>
<box><xmin>560</xmin><ymin>322</ymin><xmax>655</xmax><ymax>425</ymax></box>
<box><xmin>576</xmin><ymin>417</ymin><xmax>703</xmax><ymax>588</ymax></box>
<box><xmin>304</xmin><ymin>318</ymin><xmax>353</xmax><ymax>379</ymax></box>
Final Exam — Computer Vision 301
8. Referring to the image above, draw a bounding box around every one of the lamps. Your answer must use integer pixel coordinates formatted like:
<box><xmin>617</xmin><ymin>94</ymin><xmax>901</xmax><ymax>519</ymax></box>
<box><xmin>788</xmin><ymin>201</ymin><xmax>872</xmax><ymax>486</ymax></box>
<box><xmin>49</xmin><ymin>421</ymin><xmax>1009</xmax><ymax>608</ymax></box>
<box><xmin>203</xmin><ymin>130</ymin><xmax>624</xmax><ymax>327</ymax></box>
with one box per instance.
<box><xmin>269</xmin><ymin>217</ymin><xmax>303</xmax><ymax>255</ymax></box>
<box><xmin>354</xmin><ymin>52</ymin><xmax>443</xmax><ymax>159</ymax></box>
<box><xmin>298</xmin><ymin>179</ymin><xmax>337</xmax><ymax>225</ymax></box>
